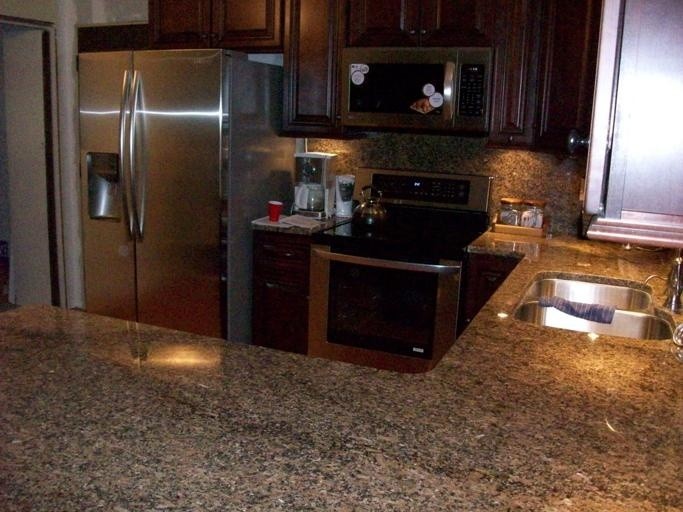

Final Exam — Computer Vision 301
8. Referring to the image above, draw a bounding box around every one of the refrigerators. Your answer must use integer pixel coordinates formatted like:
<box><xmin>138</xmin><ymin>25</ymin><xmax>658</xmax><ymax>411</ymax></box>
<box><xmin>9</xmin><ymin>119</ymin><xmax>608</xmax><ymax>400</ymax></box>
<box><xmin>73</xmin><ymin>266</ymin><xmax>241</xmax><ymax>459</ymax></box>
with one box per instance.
<box><xmin>77</xmin><ymin>46</ymin><xmax>284</xmax><ymax>343</ymax></box>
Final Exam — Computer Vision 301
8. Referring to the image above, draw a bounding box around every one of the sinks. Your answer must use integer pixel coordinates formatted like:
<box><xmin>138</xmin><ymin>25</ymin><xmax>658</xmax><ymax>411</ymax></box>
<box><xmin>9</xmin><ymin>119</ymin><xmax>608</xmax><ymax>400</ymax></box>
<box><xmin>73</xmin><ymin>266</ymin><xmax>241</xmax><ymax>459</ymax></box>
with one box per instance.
<box><xmin>510</xmin><ymin>297</ymin><xmax>676</xmax><ymax>360</ymax></box>
<box><xmin>520</xmin><ymin>267</ymin><xmax>656</xmax><ymax>314</ymax></box>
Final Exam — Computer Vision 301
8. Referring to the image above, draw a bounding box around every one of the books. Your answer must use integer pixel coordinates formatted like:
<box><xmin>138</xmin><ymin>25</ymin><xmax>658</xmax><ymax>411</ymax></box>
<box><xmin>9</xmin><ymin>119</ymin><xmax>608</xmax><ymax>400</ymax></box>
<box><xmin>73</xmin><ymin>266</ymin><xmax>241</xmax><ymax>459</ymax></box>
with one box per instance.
<box><xmin>251</xmin><ymin>213</ymin><xmax>294</xmax><ymax>230</ymax></box>
<box><xmin>279</xmin><ymin>213</ymin><xmax>327</xmax><ymax>230</ymax></box>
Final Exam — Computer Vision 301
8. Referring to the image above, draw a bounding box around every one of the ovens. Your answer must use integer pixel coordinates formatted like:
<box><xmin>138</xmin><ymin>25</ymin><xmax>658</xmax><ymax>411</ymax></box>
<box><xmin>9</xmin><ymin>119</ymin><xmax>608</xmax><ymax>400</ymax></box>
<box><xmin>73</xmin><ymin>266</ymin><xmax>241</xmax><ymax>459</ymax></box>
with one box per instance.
<box><xmin>307</xmin><ymin>240</ymin><xmax>464</xmax><ymax>375</ymax></box>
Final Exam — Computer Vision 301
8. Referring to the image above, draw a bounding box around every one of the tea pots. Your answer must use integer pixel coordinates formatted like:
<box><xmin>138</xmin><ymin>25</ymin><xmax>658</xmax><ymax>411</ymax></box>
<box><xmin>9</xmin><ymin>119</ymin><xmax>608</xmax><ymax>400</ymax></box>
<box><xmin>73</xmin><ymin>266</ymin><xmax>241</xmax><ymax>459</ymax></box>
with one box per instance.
<box><xmin>350</xmin><ymin>184</ymin><xmax>393</xmax><ymax>238</ymax></box>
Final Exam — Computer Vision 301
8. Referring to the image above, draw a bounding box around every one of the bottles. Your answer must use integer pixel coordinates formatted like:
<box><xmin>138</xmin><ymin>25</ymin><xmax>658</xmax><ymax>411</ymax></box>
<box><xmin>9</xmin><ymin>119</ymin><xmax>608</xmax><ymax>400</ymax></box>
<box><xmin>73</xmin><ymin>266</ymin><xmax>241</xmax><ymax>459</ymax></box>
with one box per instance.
<box><xmin>520</xmin><ymin>198</ymin><xmax>547</xmax><ymax>228</ymax></box>
<box><xmin>498</xmin><ymin>196</ymin><xmax>523</xmax><ymax>227</ymax></box>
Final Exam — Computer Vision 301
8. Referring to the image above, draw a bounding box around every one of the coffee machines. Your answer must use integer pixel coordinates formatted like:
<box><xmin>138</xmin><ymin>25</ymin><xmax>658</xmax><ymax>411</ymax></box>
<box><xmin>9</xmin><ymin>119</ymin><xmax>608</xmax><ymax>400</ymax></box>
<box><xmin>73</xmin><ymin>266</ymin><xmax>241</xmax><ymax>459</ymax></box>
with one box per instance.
<box><xmin>290</xmin><ymin>152</ymin><xmax>338</xmax><ymax>222</ymax></box>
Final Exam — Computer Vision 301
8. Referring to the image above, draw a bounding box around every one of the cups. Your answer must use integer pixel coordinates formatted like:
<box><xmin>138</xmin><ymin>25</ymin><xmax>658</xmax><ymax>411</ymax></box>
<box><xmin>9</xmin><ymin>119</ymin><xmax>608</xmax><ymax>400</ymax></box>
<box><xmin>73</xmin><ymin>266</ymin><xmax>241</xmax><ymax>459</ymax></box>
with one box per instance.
<box><xmin>267</xmin><ymin>200</ymin><xmax>283</xmax><ymax>223</ymax></box>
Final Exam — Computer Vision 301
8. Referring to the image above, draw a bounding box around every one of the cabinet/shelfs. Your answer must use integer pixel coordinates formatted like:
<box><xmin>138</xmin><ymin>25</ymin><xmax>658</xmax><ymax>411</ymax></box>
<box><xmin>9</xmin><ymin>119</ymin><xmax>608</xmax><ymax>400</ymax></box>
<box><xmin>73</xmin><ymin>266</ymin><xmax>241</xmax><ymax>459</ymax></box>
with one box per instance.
<box><xmin>147</xmin><ymin>1</ymin><xmax>283</xmax><ymax>52</ymax></box>
<box><xmin>277</xmin><ymin>1</ymin><xmax>366</xmax><ymax>141</ymax></box>
<box><xmin>467</xmin><ymin>254</ymin><xmax>520</xmax><ymax>324</ymax></box>
<box><xmin>250</xmin><ymin>230</ymin><xmax>307</xmax><ymax>355</ymax></box>
<box><xmin>498</xmin><ymin>1</ymin><xmax>603</xmax><ymax>158</ymax></box>
<box><xmin>348</xmin><ymin>2</ymin><xmax>500</xmax><ymax>45</ymax></box>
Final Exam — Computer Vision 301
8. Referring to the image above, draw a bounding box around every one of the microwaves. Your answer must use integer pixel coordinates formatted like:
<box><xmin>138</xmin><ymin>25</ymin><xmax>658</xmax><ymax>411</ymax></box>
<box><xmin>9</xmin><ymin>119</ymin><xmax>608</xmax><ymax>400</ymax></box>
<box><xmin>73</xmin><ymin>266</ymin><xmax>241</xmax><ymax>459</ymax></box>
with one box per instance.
<box><xmin>339</xmin><ymin>48</ymin><xmax>520</xmax><ymax>133</ymax></box>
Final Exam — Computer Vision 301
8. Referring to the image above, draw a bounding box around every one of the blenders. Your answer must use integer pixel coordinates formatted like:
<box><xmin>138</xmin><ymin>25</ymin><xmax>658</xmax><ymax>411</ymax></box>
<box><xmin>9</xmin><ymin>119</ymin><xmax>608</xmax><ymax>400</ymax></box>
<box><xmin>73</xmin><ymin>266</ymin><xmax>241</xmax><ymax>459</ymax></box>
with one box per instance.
<box><xmin>334</xmin><ymin>174</ymin><xmax>356</xmax><ymax>219</ymax></box>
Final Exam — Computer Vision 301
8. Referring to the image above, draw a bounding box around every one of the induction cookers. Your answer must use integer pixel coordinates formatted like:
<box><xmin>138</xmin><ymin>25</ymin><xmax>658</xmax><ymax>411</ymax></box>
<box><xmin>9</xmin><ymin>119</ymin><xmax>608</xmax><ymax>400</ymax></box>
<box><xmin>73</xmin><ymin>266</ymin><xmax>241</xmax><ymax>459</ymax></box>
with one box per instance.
<box><xmin>321</xmin><ymin>210</ymin><xmax>484</xmax><ymax>255</ymax></box>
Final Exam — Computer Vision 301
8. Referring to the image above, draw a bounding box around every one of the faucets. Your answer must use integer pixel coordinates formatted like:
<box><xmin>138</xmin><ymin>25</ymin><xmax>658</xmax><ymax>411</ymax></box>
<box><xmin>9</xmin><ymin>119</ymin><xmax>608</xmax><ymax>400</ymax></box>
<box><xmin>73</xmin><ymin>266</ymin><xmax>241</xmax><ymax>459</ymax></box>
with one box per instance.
<box><xmin>641</xmin><ymin>255</ymin><xmax>683</xmax><ymax>312</ymax></box>
